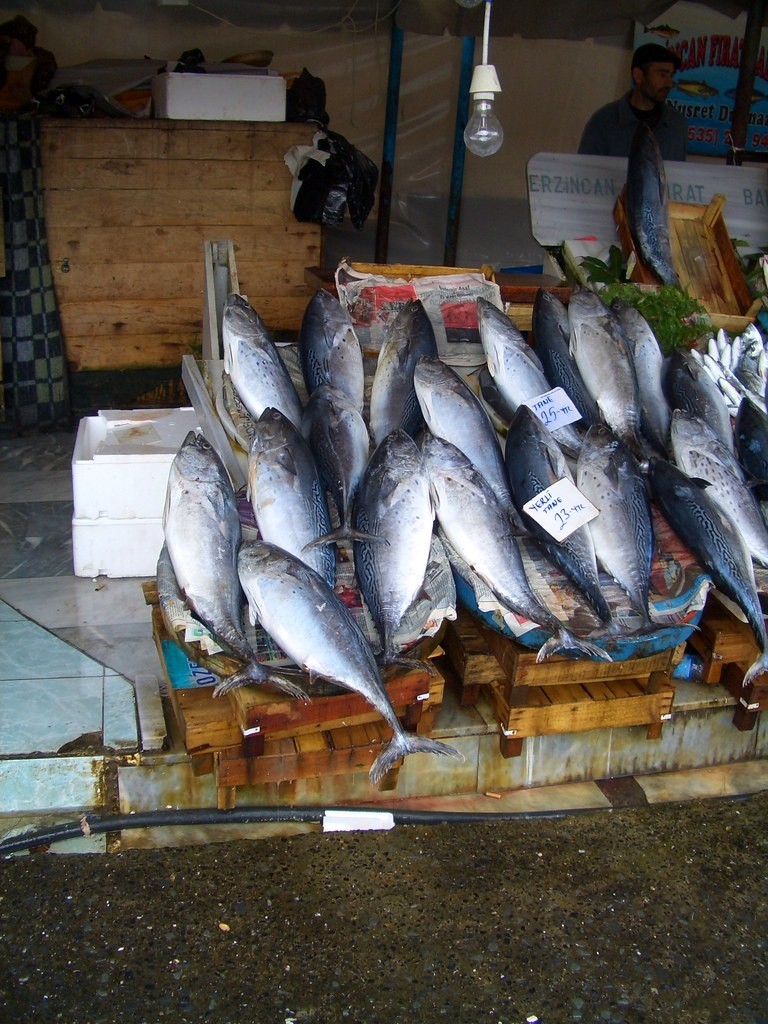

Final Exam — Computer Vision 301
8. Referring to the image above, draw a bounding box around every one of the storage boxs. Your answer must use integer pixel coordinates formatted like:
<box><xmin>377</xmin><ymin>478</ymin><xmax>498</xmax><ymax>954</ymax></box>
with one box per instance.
<box><xmin>71</xmin><ymin>518</ymin><xmax>166</xmax><ymax>580</ymax></box>
<box><xmin>612</xmin><ymin>185</ymin><xmax>764</xmax><ymax>330</ymax></box>
<box><xmin>152</xmin><ymin>70</ymin><xmax>287</xmax><ymax>123</ymax></box>
<box><xmin>71</xmin><ymin>402</ymin><xmax>196</xmax><ymax>518</ymax></box>
<box><xmin>144</xmin><ymin>569</ymin><xmax>767</xmax><ymax>815</ymax></box>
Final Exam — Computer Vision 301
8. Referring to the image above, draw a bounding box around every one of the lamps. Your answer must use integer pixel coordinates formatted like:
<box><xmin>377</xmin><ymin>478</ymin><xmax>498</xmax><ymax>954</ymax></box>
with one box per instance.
<box><xmin>463</xmin><ymin>1</ymin><xmax>505</xmax><ymax>160</ymax></box>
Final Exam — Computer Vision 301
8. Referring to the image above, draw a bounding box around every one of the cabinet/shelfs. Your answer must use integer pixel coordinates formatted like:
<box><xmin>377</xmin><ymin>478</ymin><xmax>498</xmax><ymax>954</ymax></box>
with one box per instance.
<box><xmin>38</xmin><ymin>117</ymin><xmax>326</xmax><ymax>374</ymax></box>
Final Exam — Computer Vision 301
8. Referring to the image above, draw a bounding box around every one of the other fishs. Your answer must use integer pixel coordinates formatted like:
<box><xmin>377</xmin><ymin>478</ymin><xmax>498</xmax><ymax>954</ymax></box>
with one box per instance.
<box><xmin>163</xmin><ymin>278</ymin><xmax>767</xmax><ymax>753</ymax></box>
<box><xmin>623</xmin><ymin>120</ymin><xmax>687</xmax><ymax>297</ymax></box>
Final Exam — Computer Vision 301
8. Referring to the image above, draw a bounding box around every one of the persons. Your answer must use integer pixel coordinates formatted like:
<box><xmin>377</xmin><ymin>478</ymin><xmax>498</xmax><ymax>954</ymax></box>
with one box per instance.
<box><xmin>578</xmin><ymin>41</ymin><xmax>688</xmax><ymax>164</ymax></box>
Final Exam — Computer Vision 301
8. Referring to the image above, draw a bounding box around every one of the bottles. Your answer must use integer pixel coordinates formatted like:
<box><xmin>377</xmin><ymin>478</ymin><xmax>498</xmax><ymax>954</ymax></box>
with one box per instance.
<box><xmin>671</xmin><ymin>654</ymin><xmax>704</xmax><ymax>684</ymax></box>
